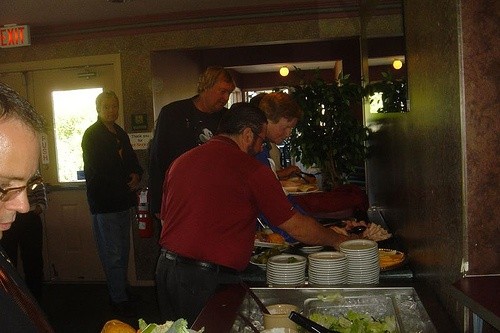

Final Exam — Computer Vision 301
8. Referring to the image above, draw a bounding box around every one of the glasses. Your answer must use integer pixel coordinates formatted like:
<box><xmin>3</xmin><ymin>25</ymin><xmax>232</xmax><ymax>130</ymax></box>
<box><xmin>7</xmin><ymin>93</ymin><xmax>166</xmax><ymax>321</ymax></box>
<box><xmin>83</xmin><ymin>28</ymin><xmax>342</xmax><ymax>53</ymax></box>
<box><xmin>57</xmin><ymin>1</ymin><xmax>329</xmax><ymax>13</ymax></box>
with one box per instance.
<box><xmin>252</xmin><ymin>130</ymin><xmax>269</xmax><ymax>147</ymax></box>
<box><xmin>0</xmin><ymin>177</ymin><xmax>42</xmax><ymax>203</ymax></box>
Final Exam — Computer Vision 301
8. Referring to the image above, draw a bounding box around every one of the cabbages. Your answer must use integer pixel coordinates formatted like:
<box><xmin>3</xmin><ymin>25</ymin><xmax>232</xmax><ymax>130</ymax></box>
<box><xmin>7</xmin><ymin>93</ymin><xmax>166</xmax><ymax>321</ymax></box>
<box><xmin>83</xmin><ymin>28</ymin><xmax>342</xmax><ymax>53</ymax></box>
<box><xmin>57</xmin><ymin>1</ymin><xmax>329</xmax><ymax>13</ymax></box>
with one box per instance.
<box><xmin>307</xmin><ymin>312</ymin><xmax>398</xmax><ymax>333</ymax></box>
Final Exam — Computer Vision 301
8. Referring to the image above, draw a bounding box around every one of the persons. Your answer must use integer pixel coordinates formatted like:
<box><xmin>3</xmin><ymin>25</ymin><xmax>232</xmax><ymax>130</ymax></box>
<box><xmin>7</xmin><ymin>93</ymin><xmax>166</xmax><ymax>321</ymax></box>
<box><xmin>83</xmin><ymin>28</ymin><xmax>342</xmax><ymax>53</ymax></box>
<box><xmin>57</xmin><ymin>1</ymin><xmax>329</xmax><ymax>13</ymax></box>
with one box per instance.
<box><xmin>82</xmin><ymin>90</ymin><xmax>143</xmax><ymax>317</ymax></box>
<box><xmin>249</xmin><ymin>93</ymin><xmax>302</xmax><ymax>179</ymax></box>
<box><xmin>0</xmin><ymin>169</ymin><xmax>48</xmax><ymax>299</ymax></box>
<box><xmin>154</xmin><ymin>102</ymin><xmax>374</xmax><ymax>329</ymax></box>
<box><xmin>0</xmin><ymin>83</ymin><xmax>57</xmax><ymax>333</ymax></box>
<box><xmin>148</xmin><ymin>64</ymin><xmax>235</xmax><ymax>220</ymax></box>
<box><xmin>252</xmin><ymin>94</ymin><xmax>309</xmax><ymax>244</ymax></box>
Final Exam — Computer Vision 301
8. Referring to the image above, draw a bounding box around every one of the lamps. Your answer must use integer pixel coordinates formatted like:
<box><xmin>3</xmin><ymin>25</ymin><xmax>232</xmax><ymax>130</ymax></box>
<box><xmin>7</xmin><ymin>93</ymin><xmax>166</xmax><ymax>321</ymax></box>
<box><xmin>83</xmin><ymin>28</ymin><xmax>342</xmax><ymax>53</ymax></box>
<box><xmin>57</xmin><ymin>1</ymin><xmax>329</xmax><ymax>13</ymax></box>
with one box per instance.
<box><xmin>279</xmin><ymin>65</ymin><xmax>289</xmax><ymax>76</ymax></box>
<box><xmin>391</xmin><ymin>58</ymin><xmax>403</xmax><ymax>69</ymax></box>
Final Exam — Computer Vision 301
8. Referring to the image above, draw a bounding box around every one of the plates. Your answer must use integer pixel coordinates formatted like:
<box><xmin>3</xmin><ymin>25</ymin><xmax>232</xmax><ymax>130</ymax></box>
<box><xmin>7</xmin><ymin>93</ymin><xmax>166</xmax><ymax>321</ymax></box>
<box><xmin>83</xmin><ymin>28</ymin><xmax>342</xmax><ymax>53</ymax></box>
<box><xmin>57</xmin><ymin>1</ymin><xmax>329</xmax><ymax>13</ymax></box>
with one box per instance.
<box><xmin>248</xmin><ymin>229</ymin><xmax>411</xmax><ymax>285</ymax></box>
<box><xmin>288</xmin><ymin>191</ymin><xmax>323</xmax><ymax>196</ymax></box>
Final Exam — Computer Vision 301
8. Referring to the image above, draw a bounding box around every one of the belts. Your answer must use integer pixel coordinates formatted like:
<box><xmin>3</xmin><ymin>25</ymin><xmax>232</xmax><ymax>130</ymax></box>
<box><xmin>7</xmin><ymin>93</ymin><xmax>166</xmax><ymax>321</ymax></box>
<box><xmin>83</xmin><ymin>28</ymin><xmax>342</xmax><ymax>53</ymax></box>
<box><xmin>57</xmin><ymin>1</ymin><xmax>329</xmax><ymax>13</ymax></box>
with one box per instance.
<box><xmin>160</xmin><ymin>250</ymin><xmax>236</xmax><ymax>277</ymax></box>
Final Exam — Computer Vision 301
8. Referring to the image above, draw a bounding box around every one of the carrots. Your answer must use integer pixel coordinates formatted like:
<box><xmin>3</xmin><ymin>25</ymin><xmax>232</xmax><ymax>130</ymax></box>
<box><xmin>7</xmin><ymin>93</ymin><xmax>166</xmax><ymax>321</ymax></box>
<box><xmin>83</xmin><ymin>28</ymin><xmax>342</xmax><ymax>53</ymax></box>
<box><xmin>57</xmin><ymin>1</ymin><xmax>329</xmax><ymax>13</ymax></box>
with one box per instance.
<box><xmin>380</xmin><ymin>251</ymin><xmax>403</xmax><ymax>268</ymax></box>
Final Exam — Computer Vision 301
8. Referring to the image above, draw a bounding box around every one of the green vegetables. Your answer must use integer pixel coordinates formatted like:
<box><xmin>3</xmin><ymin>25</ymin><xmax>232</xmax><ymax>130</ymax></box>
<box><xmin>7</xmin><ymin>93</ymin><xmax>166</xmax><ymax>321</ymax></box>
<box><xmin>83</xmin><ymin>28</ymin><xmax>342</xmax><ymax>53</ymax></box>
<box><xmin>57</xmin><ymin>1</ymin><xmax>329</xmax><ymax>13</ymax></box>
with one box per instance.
<box><xmin>252</xmin><ymin>248</ymin><xmax>283</xmax><ymax>264</ymax></box>
<box><xmin>136</xmin><ymin>317</ymin><xmax>191</xmax><ymax>333</ymax></box>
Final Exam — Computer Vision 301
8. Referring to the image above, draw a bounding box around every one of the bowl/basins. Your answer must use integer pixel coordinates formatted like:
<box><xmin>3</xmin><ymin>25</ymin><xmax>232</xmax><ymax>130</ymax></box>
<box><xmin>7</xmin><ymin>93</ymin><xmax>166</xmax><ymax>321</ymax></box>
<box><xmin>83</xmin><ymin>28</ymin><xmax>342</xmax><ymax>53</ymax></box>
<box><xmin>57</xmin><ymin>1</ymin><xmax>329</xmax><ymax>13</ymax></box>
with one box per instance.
<box><xmin>282</xmin><ymin>187</ymin><xmax>298</xmax><ymax>196</ymax></box>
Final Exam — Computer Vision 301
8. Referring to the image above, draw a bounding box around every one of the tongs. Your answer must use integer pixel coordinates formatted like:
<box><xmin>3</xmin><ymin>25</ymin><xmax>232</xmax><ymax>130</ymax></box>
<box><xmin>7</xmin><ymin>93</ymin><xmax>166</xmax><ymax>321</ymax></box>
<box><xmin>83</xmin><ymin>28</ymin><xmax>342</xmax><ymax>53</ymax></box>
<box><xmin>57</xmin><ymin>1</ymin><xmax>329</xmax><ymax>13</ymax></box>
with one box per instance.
<box><xmin>288</xmin><ymin>310</ymin><xmax>336</xmax><ymax>333</ymax></box>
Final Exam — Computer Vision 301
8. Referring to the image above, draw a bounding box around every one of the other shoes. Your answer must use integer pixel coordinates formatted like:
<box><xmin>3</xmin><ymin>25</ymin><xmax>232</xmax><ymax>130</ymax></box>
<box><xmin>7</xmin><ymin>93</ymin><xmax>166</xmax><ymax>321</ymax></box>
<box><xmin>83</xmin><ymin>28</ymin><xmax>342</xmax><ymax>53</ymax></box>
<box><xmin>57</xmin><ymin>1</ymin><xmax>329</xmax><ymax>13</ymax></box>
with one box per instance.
<box><xmin>126</xmin><ymin>292</ymin><xmax>142</xmax><ymax>303</ymax></box>
<box><xmin>33</xmin><ymin>292</ymin><xmax>41</xmax><ymax>301</ymax></box>
<box><xmin>110</xmin><ymin>302</ymin><xmax>142</xmax><ymax>319</ymax></box>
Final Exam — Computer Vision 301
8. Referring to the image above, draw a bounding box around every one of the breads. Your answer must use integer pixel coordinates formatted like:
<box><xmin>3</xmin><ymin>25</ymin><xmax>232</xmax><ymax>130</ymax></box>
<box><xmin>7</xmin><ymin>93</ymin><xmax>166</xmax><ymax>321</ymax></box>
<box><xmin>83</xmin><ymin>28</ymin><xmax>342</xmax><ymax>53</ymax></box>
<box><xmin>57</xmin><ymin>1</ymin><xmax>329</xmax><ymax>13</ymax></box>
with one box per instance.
<box><xmin>267</xmin><ymin>233</ymin><xmax>284</xmax><ymax>244</ymax></box>
<box><xmin>100</xmin><ymin>319</ymin><xmax>135</xmax><ymax>333</ymax></box>
<box><xmin>280</xmin><ymin>179</ymin><xmax>317</xmax><ymax>192</ymax></box>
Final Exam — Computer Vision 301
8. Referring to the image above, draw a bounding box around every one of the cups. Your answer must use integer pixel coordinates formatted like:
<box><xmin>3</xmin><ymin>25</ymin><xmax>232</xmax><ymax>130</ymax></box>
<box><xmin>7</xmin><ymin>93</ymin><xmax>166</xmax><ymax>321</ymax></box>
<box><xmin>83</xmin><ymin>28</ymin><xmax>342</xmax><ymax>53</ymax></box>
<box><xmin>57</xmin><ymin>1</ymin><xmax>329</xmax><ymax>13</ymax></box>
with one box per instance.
<box><xmin>261</xmin><ymin>304</ymin><xmax>303</xmax><ymax>332</ymax></box>
